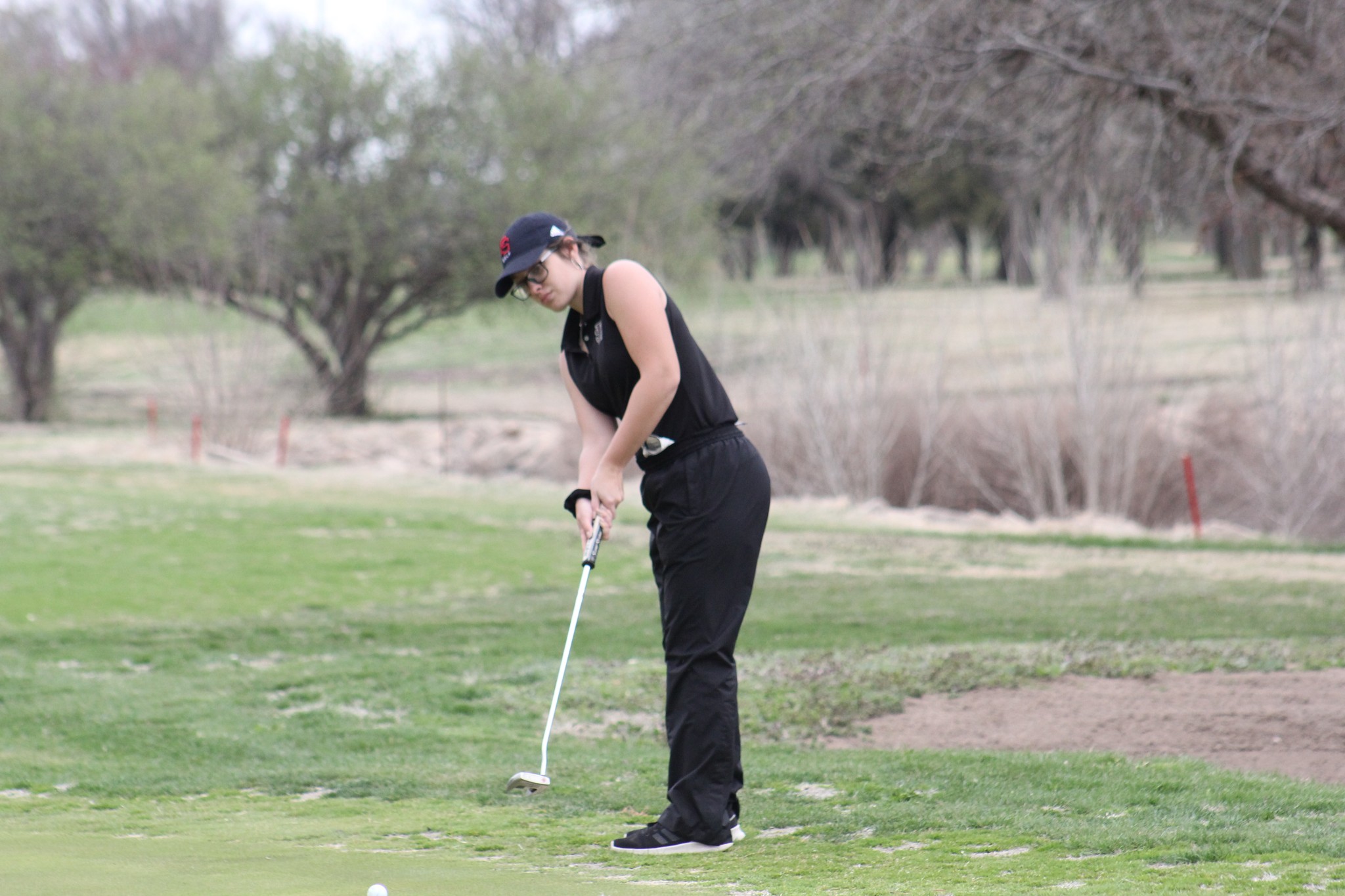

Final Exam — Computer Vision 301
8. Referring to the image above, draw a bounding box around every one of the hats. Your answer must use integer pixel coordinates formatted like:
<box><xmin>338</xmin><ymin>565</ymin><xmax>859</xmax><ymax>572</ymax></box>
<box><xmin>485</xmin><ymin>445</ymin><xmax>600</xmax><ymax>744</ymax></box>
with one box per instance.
<box><xmin>495</xmin><ymin>211</ymin><xmax>577</xmax><ymax>298</ymax></box>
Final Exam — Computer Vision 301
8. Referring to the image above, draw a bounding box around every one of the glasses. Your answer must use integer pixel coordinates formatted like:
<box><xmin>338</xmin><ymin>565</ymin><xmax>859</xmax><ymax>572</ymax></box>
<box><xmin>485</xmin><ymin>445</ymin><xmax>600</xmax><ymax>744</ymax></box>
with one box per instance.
<box><xmin>510</xmin><ymin>247</ymin><xmax>560</xmax><ymax>302</ymax></box>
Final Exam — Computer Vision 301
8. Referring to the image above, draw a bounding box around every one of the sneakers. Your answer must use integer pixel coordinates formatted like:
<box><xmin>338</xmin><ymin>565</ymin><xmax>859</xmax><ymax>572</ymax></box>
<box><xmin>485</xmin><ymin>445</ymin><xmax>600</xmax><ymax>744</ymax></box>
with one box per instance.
<box><xmin>623</xmin><ymin>806</ymin><xmax>747</xmax><ymax>841</ymax></box>
<box><xmin>611</xmin><ymin>823</ymin><xmax>734</xmax><ymax>855</ymax></box>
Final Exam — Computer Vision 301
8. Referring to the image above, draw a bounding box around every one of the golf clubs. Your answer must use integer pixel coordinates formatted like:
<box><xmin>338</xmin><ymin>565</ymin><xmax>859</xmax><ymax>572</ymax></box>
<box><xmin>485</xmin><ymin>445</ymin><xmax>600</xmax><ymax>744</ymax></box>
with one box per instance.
<box><xmin>506</xmin><ymin>510</ymin><xmax>608</xmax><ymax>792</ymax></box>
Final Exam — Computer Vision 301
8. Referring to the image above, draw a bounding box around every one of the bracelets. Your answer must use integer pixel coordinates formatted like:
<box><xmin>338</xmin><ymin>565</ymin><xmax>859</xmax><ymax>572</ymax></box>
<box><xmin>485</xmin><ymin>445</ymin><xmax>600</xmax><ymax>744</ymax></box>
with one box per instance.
<box><xmin>564</xmin><ymin>488</ymin><xmax>591</xmax><ymax>518</ymax></box>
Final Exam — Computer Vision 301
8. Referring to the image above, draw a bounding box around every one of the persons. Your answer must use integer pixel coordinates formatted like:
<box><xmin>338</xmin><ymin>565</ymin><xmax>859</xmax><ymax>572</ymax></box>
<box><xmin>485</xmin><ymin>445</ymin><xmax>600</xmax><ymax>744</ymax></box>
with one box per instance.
<box><xmin>496</xmin><ymin>215</ymin><xmax>772</xmax><ymax>858</ymax></box>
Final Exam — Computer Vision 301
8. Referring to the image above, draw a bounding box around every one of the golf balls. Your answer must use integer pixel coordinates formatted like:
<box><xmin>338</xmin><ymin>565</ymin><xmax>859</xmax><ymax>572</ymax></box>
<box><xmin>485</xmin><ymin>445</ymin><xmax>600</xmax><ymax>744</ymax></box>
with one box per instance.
<box><xmin>367</xmin><ymin>884</ymin><xmax>387</xmax><ymax>896</ymax></box>
